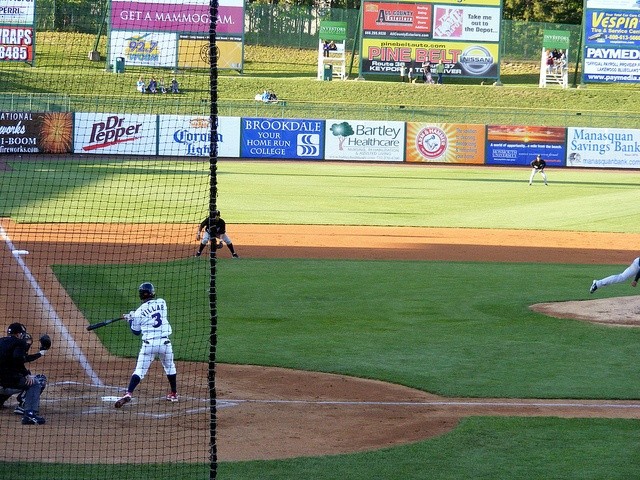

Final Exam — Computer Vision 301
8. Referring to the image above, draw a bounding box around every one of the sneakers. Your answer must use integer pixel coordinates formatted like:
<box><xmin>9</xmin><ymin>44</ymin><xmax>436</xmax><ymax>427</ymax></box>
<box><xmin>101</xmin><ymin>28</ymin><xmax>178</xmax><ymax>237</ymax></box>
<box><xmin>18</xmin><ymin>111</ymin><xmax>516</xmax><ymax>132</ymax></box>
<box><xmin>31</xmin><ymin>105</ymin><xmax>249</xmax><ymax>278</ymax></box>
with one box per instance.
<box><xmin>115</xmin><ymin>392</ymin><xmax>132</xmax><ymax>408</ymax></box>
<box><xmin>167</xmin><ymin>391</ymin><xmax>179</xmax><ymax>402</ymax></box>
<box><xmin>590</xmin><ymin>279</ymin><xmax>598</xmax><ymax>294</ymax></box>
<box><xmin>195</xmin><ymin>251</ymin><xmax>201</xmax><ymax>257</ymax></box>
<box><xmin>21</xmin><ymin>412</ymin><xmax>46</xmax><ymax>425</ymax></box>
<box><xmin>14</xmin><ymin>406</ymin><xmax>25</xmax><ymax>414</ymax></box>
<box><xmin>232</xmin><ymin>253</ymin><xmax>240</xmax><ymax>258</ymax></box>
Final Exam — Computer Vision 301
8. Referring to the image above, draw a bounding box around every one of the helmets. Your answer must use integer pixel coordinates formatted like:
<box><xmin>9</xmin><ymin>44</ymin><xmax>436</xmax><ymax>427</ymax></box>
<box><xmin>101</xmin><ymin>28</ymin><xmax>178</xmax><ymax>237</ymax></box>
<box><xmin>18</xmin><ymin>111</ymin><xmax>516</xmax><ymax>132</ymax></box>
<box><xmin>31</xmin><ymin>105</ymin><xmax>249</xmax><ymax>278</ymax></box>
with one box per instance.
<box><xmin>537</xmin><ymin>155</ymin><xmax>541</xmax><ymax>157</ymax></box>
<box><xmin>140</xmin><ymin>283</ymin><xmax>155</xmax><ymax>295</ymax></box>
<box><xmin>8</xmin><ymin>322</ymin><xmax>26</xmax><ymax>336</ymax></box>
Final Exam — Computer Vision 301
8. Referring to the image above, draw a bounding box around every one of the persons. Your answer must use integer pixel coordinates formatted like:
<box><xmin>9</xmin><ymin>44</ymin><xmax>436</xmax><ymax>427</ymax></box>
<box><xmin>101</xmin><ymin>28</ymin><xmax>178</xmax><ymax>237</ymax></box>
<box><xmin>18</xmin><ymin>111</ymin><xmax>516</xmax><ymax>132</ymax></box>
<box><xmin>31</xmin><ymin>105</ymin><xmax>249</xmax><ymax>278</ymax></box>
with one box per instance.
<box><xmin>195</xmin><ymin>209</ymin><xmax>240</xmax><ymax>259</ymax></box>
<box><xmin>435</xmin><ymin>59</ymin><xmax>445</xmax><ymax>85</ymax></box>
<box><xmin>170</xmin><ymin>78</ymin><xmax>181</xmax><ymax>93</ymax></box>
<box><xmin>407</xmin><ymin>57</ymin><xmax>415</xmax><ymax>74</ymax></box>
<box><xmin>529</xmin><ymin>154</ymin><xmax>548</xmax><ymax>186</ymax></box>
<box><xmin>86</xmin><ymin>282</ymin><xmax>178</xmax><ymax>408</ymax></box>
<box><xmin>328</xmin><ymin>40</ymin><xmax>338</xmax><ymax>51</ymax></box>
<box><xmin>136</xmin><ymin>76</ymin><xmax>146</xmax><ymax>94</ymax></box>
<box><xmin>1</xmin><ymin>322</ymin><xmax>53</xmax><ymax>425</ymax></box>
<box><xmin>148</xmin><ymin>77</ymin><xmax>158</xmax><ymax>93</ymax></box>
<box><xmin>156</xmin><ymin>76</ymin><xmax>167</xmax><ymax>94</ymax></box>
<box><xmin>260</xmin><ymin>90</ymin><xmax>271</xmax><ymax>103</ymax></box>
<box><xmin>269</xmin><ymin>89</ymin><xmax>278</xmax><ymax>103</ymax></box>
<box><xmin>399</xmin><ymin>65</ymin><xmax>405</xmax><ymax>82</ymax></box>
<box><xmin>546</xmin><ymin>48</ymin><xmax>564</xmax><ymax>75</ymax></box>
<box><xmin>421</xmin><ymin>55</ymin><xmax>431</xmax><ymax>83</ymax></box>
<box><xmin>407</xmin><ymin>67</ymin><xmax>414</xmax><ymax>83</ymax></box>
<box><xmin>589</xmin><ymin>257</ymin><xmax>640</xmax><ymax>294</ymax></box>
<box><xmin>322</xmin><ymin>40</ymin><xmax>330</xmax><ymax>57</ymax></box>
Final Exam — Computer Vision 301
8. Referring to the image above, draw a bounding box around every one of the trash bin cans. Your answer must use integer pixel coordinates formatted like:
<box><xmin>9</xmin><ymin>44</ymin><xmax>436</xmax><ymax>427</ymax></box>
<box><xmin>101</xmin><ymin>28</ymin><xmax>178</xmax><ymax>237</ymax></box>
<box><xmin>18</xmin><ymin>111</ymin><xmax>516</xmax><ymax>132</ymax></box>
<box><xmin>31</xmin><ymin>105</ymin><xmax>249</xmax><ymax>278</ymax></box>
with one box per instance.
<box><xmin>324</xmin><ymin>64</ymin><xmax>332</xmax><ymax>80</ymax></box>
<box><xmin>115</xmin><ymin>57</ymin><xmax>124</xmax><ymax>72</ymax></box>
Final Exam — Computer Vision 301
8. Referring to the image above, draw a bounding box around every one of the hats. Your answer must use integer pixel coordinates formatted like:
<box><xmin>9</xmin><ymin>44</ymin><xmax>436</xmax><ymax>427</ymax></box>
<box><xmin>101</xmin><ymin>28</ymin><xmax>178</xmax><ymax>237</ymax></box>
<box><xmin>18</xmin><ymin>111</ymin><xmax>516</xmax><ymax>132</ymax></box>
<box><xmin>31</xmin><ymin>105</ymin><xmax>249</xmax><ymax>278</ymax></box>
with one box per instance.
<box><xmin>217</xmin><ymin>211</ymin><xmax>220</xmax><ymax>216</ymax></box>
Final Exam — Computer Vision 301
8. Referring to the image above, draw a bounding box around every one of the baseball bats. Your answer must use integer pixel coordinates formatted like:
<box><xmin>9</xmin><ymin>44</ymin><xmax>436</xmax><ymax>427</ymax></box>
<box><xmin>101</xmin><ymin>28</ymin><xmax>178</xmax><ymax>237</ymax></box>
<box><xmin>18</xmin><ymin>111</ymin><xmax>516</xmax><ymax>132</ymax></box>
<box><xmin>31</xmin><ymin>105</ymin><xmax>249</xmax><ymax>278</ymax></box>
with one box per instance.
<box><xmin>86</xmin><ymin>316</ymin><xmax>125</xmax><ymax>330</ymax></box>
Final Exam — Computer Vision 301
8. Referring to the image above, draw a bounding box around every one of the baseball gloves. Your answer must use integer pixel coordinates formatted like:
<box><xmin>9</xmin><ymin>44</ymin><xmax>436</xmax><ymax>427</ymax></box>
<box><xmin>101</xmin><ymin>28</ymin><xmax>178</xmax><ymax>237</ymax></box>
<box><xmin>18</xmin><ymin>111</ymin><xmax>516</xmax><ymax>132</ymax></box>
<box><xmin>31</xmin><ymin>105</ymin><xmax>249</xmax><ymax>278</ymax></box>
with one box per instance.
<box><xmin>216</xmin><ymin>242</ymin><xmax>223</xmax><ymax>249</ymax></box>
<box><xmin>39</xmin><ymin>335</ymin><xmax>51</xmax><ymax>351</ymax></box>
